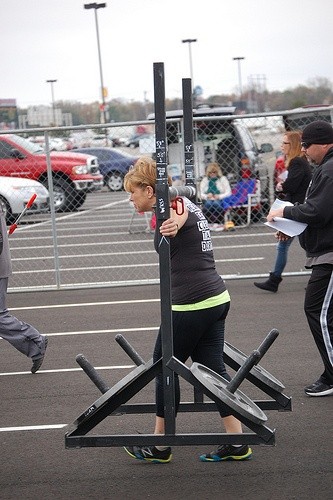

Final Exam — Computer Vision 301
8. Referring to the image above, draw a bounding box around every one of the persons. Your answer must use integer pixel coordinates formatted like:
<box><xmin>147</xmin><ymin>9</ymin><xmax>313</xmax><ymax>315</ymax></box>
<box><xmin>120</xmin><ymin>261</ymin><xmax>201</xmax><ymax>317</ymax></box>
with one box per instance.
<box><xmin>199</xmin><ymin>163</ymin><xmax>236</xmax><ymax>231</ymax></box>
<box><xmin>0</xmin><ymin>196</ymin><xmax>49</xmax><ymax>373</ymax></box>
<box><xmin>267</xmin><ymin>122</ymin><xmax>333</xmax><ymax>397</ymax></box>
<box><xmin>253</xmin><ymin>130</ymin><xmax>312</xmax><ymax>294</ymax></box>
<box><xmin>124</xmin><ymin>154</ymin><xmax>255</xmax><ymax>462</ymax></box>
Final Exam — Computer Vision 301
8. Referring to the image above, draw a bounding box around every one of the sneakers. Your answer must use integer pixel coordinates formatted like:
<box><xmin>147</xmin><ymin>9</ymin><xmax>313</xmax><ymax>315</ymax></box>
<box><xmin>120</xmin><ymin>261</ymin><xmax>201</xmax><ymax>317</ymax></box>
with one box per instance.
<box><xmin>199</xmin><ymin>444</ymin><xmax>253</xmax><ymax>462</ymax></box>
<box><xmin>304</xmin><ymin>376</ymin><xmax>333</xmax><ymax>396</ymax></box>
<box><xmin>123</xmin><ymin>445</ymin><xmax>173</xmax><ymax>463</ymax></box>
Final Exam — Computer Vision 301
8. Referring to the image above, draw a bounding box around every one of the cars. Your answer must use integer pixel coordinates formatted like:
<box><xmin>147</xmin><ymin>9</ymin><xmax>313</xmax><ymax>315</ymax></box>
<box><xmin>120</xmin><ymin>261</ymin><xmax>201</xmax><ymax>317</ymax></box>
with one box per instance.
<box><xmin>24</xmin><ymin>120</ymin><xmax>155</xmax><ymax>152</ymax></box>
<box><xmin>0</xmin><ymin>176</ymin><xmax>50</xmax><ymax>225</ymax></box>
<box><xmin>66</xmin><ymin>146</ymin><xmax>142</xmax><ymax>192</ymax></box>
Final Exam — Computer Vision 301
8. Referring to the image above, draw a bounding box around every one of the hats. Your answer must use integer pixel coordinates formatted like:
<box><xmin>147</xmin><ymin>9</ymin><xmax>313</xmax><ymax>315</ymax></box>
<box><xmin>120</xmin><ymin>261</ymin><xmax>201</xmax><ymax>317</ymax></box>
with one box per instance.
<box><xmin>301</xmin><ymin>120</ymin><xmax>333</xmax><ymax>144</ymax></box>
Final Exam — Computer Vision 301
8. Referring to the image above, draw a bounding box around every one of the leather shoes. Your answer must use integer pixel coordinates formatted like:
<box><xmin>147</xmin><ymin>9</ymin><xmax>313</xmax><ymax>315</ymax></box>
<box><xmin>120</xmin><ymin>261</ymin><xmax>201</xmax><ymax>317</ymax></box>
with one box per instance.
<box><xmin>30</xmin><ymin>336</ymin><xmax>48</xmax><ymax>373</ymax></box>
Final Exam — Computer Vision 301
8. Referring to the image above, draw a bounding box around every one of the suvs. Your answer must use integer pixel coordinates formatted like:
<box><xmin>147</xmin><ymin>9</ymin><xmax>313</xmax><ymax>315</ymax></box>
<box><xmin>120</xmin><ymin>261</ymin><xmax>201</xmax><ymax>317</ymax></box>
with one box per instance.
<box><xmin>0</xmin><ymin>133</ymin><xmax>102</xmax><ymax>215</ymax></box>
<box><xmin>144</xmin><ymin>106</ymin><xmax>274</xmax><ymax>224</ymax></box>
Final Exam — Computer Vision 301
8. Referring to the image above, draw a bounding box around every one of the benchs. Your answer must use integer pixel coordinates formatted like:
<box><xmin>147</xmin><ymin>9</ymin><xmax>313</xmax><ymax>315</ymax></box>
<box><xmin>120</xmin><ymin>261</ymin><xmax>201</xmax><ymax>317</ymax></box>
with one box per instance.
<box><xmin>225</xmin><ymin>179</ymin><xmax>261</xmax><ymax>228</ymax></box>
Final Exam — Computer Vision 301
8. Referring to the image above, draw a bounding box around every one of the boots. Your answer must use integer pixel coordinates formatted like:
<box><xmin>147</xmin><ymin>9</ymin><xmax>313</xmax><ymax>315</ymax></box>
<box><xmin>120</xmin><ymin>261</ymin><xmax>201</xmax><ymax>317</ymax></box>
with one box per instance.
<box><xmin>254</xmin><ymin>272</ymin><xmax>283</xmax><ymax>292</ymax></box>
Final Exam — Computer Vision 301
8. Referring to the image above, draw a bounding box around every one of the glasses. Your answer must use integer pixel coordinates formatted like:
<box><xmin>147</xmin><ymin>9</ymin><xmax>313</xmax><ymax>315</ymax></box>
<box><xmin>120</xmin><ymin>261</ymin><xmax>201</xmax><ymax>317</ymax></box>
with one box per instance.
<box><xmin>301</xmin><ymin>141</ymin><xmax>312</xmax><ymax>149</ymax></box>
<box><xmin>281</xmin><ymin>142</ymin><xmax>290</xmax><ymax>144</ymax></box>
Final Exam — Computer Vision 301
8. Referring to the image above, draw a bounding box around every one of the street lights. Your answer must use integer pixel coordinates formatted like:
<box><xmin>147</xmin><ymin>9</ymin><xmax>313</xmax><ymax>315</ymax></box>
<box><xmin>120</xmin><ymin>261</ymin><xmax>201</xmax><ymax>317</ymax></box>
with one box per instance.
<box><xmin>47</xmin><ymin>79</ymin><xmax>57</xmax><ymax>126</ymax></box>
<box><xmin>84</xmin><ymin>2</ymin><xmax>107</xmax><ymax>147</ymax></box>
<box><xmin>232</xmin><ymin>56</ymin><xmax>245</xmax><ymax>112</ymax></box>
<box><xmin>183</xmin><ymin>39</ymin><xmax>196</xmax><ymax>78</ymax></box>
